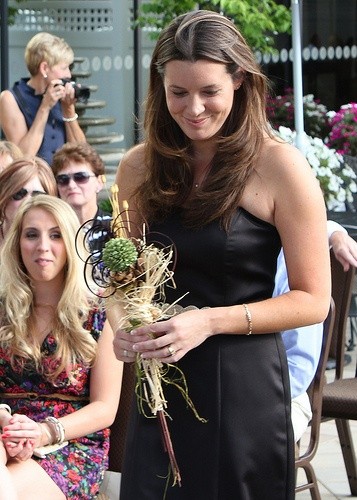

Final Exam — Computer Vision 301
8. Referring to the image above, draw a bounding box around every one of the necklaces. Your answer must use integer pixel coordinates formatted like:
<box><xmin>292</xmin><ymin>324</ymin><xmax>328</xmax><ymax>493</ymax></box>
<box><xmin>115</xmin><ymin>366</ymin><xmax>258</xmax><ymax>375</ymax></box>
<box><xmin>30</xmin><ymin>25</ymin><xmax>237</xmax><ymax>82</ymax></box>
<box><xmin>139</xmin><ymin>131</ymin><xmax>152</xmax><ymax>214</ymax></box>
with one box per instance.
<box><xmin>194</xmin><ymin>159</ymin><xmax>210</xmax><ymax>187</ymax></box>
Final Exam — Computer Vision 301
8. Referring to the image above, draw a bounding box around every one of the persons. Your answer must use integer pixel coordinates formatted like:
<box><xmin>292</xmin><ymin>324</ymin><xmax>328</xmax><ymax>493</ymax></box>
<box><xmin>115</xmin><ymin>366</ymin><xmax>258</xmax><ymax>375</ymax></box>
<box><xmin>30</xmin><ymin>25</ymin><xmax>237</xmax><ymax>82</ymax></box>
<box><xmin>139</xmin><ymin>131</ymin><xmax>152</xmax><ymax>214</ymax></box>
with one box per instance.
<box><xmin>272</xmin><ymin>220</ymin><xmax>357</xmax><ymax>443</ymax></box>
<box><xmin>0</xmin><ymin>140</ymin><xmax>114</xmax><ymax>248</ymax></box>
<box><xmin>0</xmin><ymin>32</ymin><xmax>86</xmax><ymax>167</ymax></box>
<box><xmin>0</xmin><ymin>194</ymin><xmax>124</xmax><ymax>500</ymax></box>
<box><xmin>102</xmin><ymin>10</ymin><xmax>331</xmax><ymax>500</ymax></box>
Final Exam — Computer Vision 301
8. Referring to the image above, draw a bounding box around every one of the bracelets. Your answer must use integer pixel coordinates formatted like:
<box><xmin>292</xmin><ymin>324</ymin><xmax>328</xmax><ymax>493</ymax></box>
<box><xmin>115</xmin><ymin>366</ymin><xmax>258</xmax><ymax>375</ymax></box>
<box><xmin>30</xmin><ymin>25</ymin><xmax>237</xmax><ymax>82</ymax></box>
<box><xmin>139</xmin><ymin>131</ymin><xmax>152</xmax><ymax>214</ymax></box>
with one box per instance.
<box><xmin>62</xmin><ymin>113</ymin><xmax>78</xmax><ymax>122</ymax></box>
<box><xmin>243</xmin><ymin>304</ymin><xmax>252</xmax><ymax>335</ymax></box>
<box><xmin>0</xmin><ymin>404</ymin><xmax>11</xmax><ymax>415</ymax></box>
<box><xmin>37</xmin><ymin>415</ymin><xmax>65</xmax><ymax>447</ymax></box>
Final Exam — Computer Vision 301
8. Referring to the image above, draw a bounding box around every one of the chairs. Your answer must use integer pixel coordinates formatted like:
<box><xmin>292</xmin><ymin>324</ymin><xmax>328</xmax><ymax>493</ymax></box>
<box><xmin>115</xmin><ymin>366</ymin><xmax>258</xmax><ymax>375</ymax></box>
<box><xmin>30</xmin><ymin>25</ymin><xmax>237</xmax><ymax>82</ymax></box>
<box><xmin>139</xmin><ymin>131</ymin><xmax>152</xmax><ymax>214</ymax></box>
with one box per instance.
<box><xmin>98</xmin><ymin>362</ymin><xmax>136</xmax><ymax>500</ymax></box>
<box><xmin>294</xmin><ymin>225</ymin><xmax>357</xmax><ymax>500</ymax></box>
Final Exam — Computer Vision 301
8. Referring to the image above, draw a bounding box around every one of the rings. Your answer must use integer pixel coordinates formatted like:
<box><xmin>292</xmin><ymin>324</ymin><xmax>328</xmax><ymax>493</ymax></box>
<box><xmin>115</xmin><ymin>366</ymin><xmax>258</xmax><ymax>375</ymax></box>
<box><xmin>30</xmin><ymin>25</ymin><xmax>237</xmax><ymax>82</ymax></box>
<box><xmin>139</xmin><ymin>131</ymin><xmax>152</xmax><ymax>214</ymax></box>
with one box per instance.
<box><xmin>124</xmin><ymin>350</ymin><xmax>128</xmax><ymax>357</ymax></box>
<box><xmin>168</xmin><ymin>346</ymin><xmax>175</xmax><ymax>355</ymax></box>
<box><xmin>56</xmin><ymin>91</ymin><xmax>60</xmax><ymax>95</ymax></box>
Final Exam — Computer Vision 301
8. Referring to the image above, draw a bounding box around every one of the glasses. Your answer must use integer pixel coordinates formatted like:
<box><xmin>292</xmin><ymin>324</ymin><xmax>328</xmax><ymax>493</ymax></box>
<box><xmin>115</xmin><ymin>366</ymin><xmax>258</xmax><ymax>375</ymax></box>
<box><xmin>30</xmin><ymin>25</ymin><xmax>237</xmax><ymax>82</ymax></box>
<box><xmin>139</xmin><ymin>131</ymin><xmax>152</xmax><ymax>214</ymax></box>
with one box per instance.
<box><xmin>13</xmin><ymin>187</ymin><xmax>46</xmax><ymax>200</ymax></box>
<box><xmin>56</xmin><ymin>172</ymin><xmax>97</xmax><ymax>184</ymax></box>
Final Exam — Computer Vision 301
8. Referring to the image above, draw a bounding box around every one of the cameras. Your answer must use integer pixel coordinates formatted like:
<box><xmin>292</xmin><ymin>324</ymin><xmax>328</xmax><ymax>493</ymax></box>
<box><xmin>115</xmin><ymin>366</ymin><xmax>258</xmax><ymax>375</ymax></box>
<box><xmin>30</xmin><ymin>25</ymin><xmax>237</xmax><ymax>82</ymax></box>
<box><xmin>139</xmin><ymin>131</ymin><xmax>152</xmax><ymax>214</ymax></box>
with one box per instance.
<box><xmin>55</xmin><ymin>78</ymin><xmax>90</xmax><ymax>103</ymax></box>
